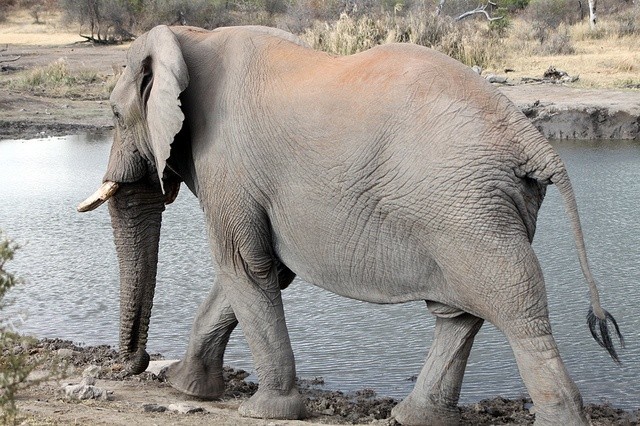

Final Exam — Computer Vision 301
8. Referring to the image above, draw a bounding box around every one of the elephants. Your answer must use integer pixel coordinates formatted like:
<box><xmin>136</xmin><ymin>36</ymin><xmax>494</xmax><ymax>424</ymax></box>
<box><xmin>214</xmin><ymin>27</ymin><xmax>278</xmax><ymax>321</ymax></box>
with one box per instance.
<box><xmin>77</xmin><ymin>24</ymin><xmax>625</xmax><ymax>426</ymax></box>
